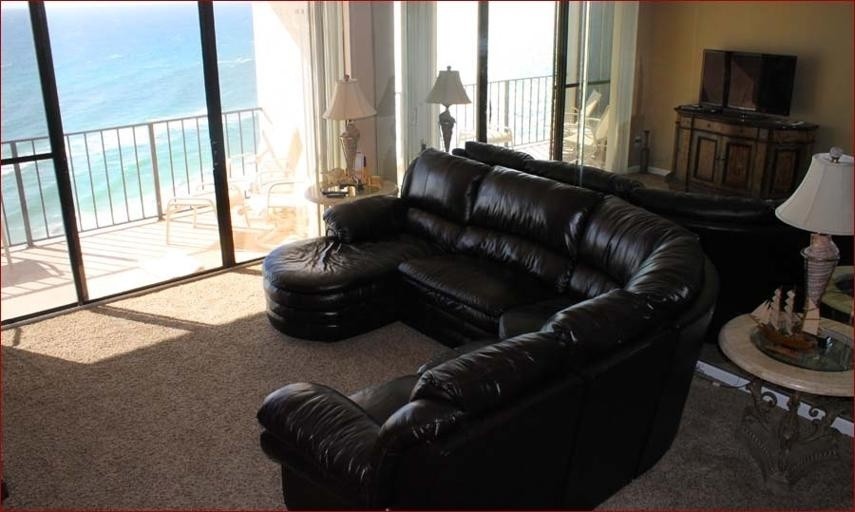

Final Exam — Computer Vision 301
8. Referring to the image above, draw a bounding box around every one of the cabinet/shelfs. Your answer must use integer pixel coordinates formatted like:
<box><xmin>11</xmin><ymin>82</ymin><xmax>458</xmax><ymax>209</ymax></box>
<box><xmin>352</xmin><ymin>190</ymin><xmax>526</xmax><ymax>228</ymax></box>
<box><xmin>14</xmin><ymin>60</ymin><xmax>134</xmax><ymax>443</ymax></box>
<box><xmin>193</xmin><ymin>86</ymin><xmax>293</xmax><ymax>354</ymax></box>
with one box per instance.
<box><xmin>664</xmin><ymin>105</ymin><xmax>820</xmax><ymax>198</ymax></box>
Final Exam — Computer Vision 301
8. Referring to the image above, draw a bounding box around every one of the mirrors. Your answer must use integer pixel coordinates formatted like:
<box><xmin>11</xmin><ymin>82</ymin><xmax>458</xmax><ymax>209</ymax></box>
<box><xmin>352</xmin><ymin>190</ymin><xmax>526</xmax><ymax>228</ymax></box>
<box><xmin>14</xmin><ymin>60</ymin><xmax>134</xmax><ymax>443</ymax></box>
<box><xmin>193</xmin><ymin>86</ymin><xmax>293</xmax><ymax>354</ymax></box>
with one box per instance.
<box><xmin>393</xmin><ymin>0</ymin><xmax>855</xmax><ymax>440</ymax></box>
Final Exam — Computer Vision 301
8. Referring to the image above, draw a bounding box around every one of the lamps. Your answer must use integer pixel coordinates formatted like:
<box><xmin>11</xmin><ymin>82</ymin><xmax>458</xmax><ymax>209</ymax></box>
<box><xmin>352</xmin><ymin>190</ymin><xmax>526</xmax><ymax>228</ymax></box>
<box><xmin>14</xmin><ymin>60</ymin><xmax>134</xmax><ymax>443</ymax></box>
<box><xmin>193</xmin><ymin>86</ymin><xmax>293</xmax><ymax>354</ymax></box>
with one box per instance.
<box><xmin>752</xmin><ymin>146</ymin><xmax>855</xmax><ymax>372</ymax></box>
<box><xmin>322</xmin><ymin>75</ymin><xmax>380</xmax><ymax>195</ymax></box>
<box><xmin>425</xmin><ymin>66</ymin><xmax>471</xmax><ymax>152</ymax></box>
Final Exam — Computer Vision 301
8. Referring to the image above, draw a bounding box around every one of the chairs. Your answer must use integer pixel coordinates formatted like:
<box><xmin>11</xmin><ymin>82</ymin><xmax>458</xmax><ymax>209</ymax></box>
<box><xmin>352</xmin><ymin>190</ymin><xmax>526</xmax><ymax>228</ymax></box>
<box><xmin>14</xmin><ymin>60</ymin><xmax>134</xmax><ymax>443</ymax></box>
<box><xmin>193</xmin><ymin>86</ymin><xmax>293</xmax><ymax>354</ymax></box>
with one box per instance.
<box><xmin>562</xmin><ymin>89</ymin><xmax>610</xmax><ymax>162</ymax></box>
<box><xmin>165</xmin><ymin>119</ymin><xmax>302</xmax><ymax>247</ymax></box>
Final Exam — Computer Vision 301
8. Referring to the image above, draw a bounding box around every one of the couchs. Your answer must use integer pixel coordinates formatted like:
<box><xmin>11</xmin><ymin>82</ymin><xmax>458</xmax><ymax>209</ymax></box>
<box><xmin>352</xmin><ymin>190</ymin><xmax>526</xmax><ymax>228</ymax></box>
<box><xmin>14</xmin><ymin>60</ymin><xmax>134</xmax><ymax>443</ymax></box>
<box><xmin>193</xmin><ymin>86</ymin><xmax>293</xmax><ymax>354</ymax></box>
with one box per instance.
<box><xmin>258</xmin><ymin>150</ymin><xmax>717</xmax><ymax>512</ymax></box>
<box><xmin>452</xmin><ymin>141</ymin><xmax>814</xmax><ymax>340</ymax></box>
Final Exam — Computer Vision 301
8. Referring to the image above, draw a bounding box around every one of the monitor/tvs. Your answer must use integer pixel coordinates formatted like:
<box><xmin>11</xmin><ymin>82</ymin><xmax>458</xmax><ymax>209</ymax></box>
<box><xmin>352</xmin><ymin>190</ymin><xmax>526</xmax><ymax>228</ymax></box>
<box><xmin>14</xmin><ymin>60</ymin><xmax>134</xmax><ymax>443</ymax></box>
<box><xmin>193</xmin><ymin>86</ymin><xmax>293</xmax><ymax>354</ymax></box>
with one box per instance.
<box><xmin>698</xmin><ymin>48</ymin><xmax>797</xmax><ymax>122</ymax></box>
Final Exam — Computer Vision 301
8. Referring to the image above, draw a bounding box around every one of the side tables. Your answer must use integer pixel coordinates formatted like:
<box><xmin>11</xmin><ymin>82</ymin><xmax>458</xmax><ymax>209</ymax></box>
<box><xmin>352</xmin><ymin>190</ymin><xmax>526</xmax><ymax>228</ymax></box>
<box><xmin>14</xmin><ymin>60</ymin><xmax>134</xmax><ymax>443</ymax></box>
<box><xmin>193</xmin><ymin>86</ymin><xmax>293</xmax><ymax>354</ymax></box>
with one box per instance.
<box><xmin>820</xmin><ymin>265</ymin><xmax>855</xmax><ymax>316</ymax></box>
<box><xmin>720</xmin><ymin>308</ymin><xmax>855</xmax><ymax>486</ymax></box>
<box><xmin>304</xmin><ymin>181</ymin><xmax>398</xmax><ymax>236</ymax></box>
